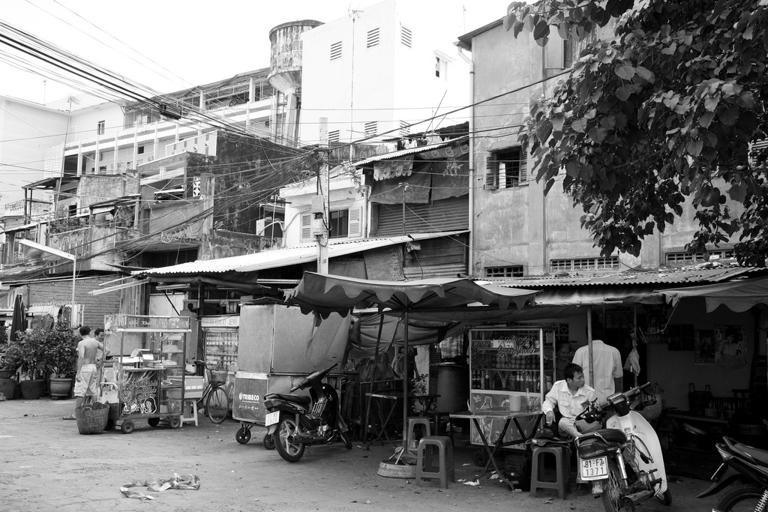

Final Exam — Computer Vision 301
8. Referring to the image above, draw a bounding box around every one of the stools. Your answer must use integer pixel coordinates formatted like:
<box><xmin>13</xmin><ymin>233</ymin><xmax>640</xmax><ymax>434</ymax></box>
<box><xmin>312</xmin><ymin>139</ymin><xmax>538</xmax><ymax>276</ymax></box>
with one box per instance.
<box><xmin>173</xmin><ymin>398</ymin><xmax>199</xmax><ymax>428</ymax></box>
<box><xmin>528</xmin><ymin>443</ymin><xmax>572</xmax><ymax>500</ymax></box>
<box><xmin>407</xmin><ymin>417</ymin><xmax>456</xmax><ymax>490</ymax></box>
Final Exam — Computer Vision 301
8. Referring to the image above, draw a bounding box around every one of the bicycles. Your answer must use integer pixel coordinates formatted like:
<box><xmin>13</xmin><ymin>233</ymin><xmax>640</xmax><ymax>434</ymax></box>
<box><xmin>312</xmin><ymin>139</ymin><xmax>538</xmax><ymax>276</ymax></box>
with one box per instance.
<box><xmin>194</xmin><ymin>358</ymin><xmax>230</xmax><ymax>425</ymax></box>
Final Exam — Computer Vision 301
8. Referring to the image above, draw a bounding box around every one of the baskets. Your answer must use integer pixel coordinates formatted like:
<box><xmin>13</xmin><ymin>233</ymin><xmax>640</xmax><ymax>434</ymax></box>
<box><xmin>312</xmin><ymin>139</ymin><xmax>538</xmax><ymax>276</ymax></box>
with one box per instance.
<box><xmin>75</xmin><ymin>401</ymin><xmax>110</xmax><ymax>435</ymax></box>
<box><xmin>206</xmin><ymin>369</ymin><xmax>228</xmax><ymax>384</ymax></box>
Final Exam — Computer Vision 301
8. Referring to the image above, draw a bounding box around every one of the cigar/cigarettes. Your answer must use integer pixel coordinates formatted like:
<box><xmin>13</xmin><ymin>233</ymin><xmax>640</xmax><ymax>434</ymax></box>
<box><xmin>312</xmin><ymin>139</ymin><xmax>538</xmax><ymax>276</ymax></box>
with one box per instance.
<box><xmin>545</xmin><ymin>421</ymin><xmax>550</xmax><ymax>424</ymax></box>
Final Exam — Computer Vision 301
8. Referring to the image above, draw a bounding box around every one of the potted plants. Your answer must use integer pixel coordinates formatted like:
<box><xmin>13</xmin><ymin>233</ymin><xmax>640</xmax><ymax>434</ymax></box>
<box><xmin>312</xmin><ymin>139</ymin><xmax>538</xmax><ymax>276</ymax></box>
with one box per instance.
<box><xmin>0</xmin><ymin>325</ymin><xmax>81</xmax><ymax>398</ymax></box>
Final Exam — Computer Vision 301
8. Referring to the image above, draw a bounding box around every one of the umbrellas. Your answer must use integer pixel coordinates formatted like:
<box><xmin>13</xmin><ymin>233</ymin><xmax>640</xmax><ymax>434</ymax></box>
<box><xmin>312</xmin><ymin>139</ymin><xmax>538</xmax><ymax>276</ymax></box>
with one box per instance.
<box><xmin>10</xmin><ymin>293</ymin><xmax>25</xmax><ymax>342</ymax></box>
<box><xmin>278</xmin><ymin>269</ymin><xmax>546</xmax><ymax>453</ymax></box>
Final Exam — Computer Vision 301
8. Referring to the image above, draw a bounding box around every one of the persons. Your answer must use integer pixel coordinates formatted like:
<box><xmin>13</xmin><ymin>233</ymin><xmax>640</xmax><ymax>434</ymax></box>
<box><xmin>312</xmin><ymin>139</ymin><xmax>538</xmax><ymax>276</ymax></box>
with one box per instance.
<box><xmin>541</xmin><ymin>362</ymin><xmax>605</xmax><ymax>499</ymax></box>
<box><xmin>545</xmin><ymin>341</ymin><xmax>573</xmax><ymax>392</ymax></box>
<box><xmin>81</xmin><ymin>328</ymin><xmax>106</xmax><ymax>407</ymax></box>
<box><xmin>62</xmin><ymin>326</ymin><xmax>111</xmax><ymax>419</ymax></box>
<box><xmin>571</xmin><ymin>321</ymin><xmax>625</xmax><ymax>405</ymax></box>
<box><xmin>344</xmin><ymin>346</ymin><xmax>425</xmax><ymax>383</ymax></box>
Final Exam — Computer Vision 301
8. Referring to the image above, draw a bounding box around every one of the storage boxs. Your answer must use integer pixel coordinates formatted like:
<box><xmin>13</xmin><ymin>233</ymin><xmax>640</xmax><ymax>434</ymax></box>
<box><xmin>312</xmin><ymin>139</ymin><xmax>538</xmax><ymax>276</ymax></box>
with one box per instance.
<box><xmin>167</xmin><ymin>375</ymin><xmax>204</xmax><ymax>399</ymax></box>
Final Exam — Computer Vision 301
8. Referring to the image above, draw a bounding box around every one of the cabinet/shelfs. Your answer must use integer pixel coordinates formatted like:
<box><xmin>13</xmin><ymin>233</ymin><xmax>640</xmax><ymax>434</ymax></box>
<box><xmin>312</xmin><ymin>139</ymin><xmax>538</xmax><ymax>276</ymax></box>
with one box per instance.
<box><xmin>200</xmin><ymin>327</ymin><xmax>239</xmax><ymax>409</ymax></box>
<box><xmin>468</xmin><ymin>326</ymin><xmax>557</xmax><ymax>451</ymax></box>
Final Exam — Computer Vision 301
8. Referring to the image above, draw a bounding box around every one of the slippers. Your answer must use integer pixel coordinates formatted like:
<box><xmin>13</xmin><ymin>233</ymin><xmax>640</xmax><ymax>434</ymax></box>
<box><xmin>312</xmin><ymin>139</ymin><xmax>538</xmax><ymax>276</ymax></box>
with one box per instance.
<box><xmin>63</xmin><ymin>415</ymin><xmax>76</xmax><ymax>420</ymax></box>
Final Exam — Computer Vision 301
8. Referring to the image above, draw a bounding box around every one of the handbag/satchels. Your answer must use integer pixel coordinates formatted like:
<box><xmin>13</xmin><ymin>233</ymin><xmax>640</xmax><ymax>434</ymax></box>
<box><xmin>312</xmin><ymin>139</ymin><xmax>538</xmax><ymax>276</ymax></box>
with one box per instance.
<box><xmin>532</xmin><ymin>430</ymin><xmax>574</xmax><ymax>448</ymax></box>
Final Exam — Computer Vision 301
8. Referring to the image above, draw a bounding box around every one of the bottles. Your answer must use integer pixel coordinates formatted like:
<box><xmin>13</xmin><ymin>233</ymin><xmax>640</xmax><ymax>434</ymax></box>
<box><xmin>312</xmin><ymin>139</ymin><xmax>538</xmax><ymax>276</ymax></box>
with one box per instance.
<box><xmin>515</xmin><ymin>371</ymin><xmax>540</xmax><ymax>392</ymax></box>
<box><xmin>471</xmin><ymin>369</ymin><xmax>487</xmax><ymax>390</ymax></box>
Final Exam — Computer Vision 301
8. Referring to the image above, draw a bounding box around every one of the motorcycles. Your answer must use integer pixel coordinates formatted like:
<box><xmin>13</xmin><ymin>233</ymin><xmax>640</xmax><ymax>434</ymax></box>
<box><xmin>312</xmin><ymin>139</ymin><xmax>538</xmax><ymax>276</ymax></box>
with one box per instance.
<box><xmin>572</xmin><ymin>380</ymin><xmax>672</xmax><ymax>512</ymax></box>
<box><xmin>263</xmin><ymin>361</ymin><xmax>353</xmax><ymax>464</ymax></box>
<box><xmin>695</xmin><ymin>435</ymin><xmax>768</xmax><ymax>512</ymax></box>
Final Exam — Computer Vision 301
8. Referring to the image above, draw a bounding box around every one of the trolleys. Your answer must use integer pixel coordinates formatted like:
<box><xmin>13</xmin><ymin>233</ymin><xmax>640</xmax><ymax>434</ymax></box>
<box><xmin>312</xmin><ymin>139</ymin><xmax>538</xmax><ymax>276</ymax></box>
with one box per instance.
<box><xmin>231</xmin><ymin>294</ymin><xmax>362</xmax><ymax>451</ymax></box>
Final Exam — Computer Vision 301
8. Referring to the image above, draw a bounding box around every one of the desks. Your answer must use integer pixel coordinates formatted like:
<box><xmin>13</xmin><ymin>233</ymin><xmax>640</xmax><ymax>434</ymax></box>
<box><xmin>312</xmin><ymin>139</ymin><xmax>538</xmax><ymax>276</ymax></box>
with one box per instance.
<box><xmin>450</xmin><ymin>407</ymin><xmax>544</xmax><ymax>491</ymax></box>
<box><xmin>365</xmin><ymin>391</ymin><xmax>441</xmax><ymax>451</ymax></box>
